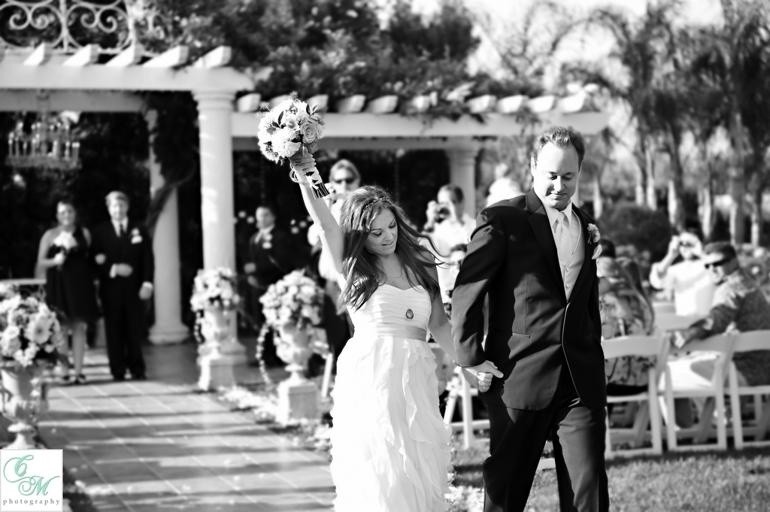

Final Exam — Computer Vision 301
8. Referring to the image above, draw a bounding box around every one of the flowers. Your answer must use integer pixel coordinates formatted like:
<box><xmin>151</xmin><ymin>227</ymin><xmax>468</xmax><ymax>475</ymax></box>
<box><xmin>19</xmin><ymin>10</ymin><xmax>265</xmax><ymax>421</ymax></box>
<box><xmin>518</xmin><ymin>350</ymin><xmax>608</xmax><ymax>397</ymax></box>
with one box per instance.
<box><xmin>257</xmin><ymin>100</ymin><xmax>332</xmax><ymax>200</ymax></box>
<box><xmin>258</xmin><ymin>269</ymin><xmax>326</xmax><ymax>336</ymax></box>
<box><xmin>188</xmin><ymin>264</ymin><xmax>243</xmax><ymax>317</ymax></box>
<box><xmin>1</xmin><ymin>282</ymin><xmax>75</xmax><ymax>374</ymax></box>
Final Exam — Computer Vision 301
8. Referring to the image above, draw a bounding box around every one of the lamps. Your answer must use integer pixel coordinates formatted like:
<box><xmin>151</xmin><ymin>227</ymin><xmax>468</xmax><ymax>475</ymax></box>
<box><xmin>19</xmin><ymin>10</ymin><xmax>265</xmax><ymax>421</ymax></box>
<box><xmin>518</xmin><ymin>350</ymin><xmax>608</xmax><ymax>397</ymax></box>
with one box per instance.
<box><xmin>5</xmin><ymin>90</ymin><xmax>83</xmax><ymax>172</ymax></box>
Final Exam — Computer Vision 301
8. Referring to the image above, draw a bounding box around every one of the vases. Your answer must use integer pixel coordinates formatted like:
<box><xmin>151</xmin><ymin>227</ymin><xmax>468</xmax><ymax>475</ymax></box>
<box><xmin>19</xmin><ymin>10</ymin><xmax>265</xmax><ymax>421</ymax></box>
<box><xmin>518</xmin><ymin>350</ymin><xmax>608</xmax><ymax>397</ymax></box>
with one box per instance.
<box><xmin>201</xmin><ymin>310</ymin><xmax>229</xmax><ymax>339</ymax></box>
<box><xmin>0</xmin><ymin>361</ymin><xmax>50</xmax><ymax>396</ymax></box>
<box><xmin>280</xmin><ymin>327</ymin><xmax>314</xmax><ymax>347</ymax></box>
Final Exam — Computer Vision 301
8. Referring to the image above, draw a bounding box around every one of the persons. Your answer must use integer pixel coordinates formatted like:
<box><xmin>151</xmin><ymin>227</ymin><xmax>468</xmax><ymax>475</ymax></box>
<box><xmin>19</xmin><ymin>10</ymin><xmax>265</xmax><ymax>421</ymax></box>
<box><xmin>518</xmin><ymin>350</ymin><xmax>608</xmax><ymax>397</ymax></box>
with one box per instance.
<box><xmin>36</xmin><ymin>198</ymin><xmax>95</xmax><ymax>387</ymax></box>
<box><xmin>585</xmin><ymin>237</ymin><xmax>656</xmax><ymax>395</ymax></box>
<box><xmin>85</xmin><ymin>190</ymin><xmax>156</xmax><ymax>383</ymax></box>
<box><xmin>416</xmin><ymin>182</ymin><xmax>480</xmax><ymax>306</ymax></box>
<box><xmin>241</xmin><ymin>202</ymin><xmax>296</xmax><ymax>368</ymax></box>
<box><xmin>662</xmin><ymin>240</ymin><xmax>769</xmax><ymax>390</ymax></box>
<box><xmin>640</xmin><ymin>220</ymin><xmax>721</xmax><ymax>318</ymax></box>
<box><xmin>305</xmin><ymin>158</ymin><xmax>364</xmax><ymax>361</ymax></box>
<box><xmin>280</xmin><ymin>141</ymin><xmax>501</xmax><ymax>512</ymax></box>
<box><xmin>451</xmin><ymin>121</ymin><xmax>615</xmax><ymax>512</ymax></box>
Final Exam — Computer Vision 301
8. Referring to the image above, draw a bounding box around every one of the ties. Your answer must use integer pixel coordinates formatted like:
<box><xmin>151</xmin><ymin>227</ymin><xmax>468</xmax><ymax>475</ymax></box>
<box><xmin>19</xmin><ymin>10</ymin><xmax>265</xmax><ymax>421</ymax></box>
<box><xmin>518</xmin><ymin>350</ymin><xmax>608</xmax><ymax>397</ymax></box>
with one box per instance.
<box><xmin>120</xmin><ymin>223</ymin><xmax>125</xmax><ymax>240</ymax></box>
<box><xmin>554</xmin><ymin>212</ymin><xmax>571</xmax><ymax>270</ymax></box>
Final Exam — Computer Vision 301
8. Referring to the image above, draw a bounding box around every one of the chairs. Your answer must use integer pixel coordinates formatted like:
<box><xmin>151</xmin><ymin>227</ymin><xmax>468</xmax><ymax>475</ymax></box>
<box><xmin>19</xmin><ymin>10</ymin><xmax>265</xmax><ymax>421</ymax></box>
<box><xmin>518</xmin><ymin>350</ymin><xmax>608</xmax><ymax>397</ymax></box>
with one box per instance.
<box><xmin>444</xmin><ymin>286</ymin><xmax>770</xmax><ymax>466</ymax></box>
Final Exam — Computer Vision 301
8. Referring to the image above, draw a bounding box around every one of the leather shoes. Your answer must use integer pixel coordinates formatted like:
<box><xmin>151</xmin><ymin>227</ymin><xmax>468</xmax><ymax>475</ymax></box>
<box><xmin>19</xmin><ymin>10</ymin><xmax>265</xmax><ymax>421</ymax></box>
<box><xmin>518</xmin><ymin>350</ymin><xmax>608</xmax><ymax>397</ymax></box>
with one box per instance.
<box><xmin>112</xmin><ymin>371</ymin><xmax>147</xmax><ymax>382</ymax></box>
<box><xmin>60</xmin><ymin>365</ymin><xmax>84</xmax><ymax>385</ymax></box>
